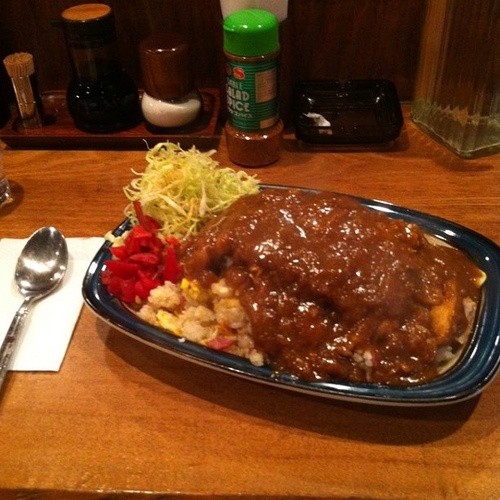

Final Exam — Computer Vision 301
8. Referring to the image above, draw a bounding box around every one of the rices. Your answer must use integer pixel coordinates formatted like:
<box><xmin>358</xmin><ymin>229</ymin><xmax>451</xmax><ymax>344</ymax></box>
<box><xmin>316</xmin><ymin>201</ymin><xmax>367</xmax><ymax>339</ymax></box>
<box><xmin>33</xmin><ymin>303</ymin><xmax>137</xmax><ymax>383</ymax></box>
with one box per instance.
<box><xmin>137</xmin><ymin>276</ymin><xmax>266</xmax><ymax>367</ymax></box>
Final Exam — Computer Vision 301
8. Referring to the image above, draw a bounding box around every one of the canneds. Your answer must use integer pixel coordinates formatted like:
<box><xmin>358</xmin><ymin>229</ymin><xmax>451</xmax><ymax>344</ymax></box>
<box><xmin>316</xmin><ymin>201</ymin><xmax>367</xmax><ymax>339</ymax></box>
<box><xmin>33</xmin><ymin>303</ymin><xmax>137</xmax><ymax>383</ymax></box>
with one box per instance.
<box><xmin>220</xmin><ymin>8</ymin><xmax>284</xmax><ymax>167</ymax></box>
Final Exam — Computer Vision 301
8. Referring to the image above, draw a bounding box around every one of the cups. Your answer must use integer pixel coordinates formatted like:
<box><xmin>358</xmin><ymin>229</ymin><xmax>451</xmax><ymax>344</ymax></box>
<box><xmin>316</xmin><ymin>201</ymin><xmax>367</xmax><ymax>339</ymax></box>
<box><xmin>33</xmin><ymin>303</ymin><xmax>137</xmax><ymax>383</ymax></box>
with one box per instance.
<box><xmin>9</xmin><ymin>71</ymin><xmax>44</xmax><ymax>120</ymax></box>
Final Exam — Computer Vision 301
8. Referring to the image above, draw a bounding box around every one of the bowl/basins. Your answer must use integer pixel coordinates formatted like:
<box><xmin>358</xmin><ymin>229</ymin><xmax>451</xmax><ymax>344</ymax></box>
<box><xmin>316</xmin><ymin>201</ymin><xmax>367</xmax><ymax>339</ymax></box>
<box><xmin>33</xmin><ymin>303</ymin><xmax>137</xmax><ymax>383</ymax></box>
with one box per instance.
<box><xmin>292</xmin><ymin>79</ymin><xmax>402</xmax><ymax>144</ymax></box>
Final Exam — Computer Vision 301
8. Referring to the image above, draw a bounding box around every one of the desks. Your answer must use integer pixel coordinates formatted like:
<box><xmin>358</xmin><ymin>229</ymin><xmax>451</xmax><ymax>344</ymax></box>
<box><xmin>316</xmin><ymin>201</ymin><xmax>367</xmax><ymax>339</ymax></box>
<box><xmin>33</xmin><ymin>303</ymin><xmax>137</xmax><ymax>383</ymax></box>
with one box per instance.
<box><xmin>0</xmin><ymin>103</ymin><xmax>500</xmax><ymax>500</ymax></box>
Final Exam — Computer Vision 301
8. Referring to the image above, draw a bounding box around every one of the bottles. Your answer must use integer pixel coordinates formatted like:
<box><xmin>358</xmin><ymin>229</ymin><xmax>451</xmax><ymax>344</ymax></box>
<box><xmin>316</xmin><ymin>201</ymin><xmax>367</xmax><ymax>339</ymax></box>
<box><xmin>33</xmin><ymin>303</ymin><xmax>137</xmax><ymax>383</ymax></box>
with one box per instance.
<box><xmin>409</xmin><ymin>0</ymin><xmax>499</xmax><ymax>158</ymax></box>
<box><xmin>138</xmin><ymin>33</ymin><xmax>203</xmax><ymax>128</ymax></box>
<box><xmin>57</xmin><ymin>3</ymin><xmax>139</xmax><ymax>130</ymax></box>
<box><xmin>222</xmin><ymin>11</ymin><xmax>284</xmax><ymax>166</ymax></box>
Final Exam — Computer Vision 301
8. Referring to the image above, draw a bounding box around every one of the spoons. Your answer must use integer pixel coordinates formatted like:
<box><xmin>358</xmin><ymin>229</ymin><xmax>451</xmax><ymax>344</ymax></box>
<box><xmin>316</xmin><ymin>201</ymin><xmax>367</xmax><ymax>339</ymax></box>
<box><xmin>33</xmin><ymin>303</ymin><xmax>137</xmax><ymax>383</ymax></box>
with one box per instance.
<box><xmin>0</xmin><ymin>225</ymin><xmax>69</xmax><ymax>388</ymax></box>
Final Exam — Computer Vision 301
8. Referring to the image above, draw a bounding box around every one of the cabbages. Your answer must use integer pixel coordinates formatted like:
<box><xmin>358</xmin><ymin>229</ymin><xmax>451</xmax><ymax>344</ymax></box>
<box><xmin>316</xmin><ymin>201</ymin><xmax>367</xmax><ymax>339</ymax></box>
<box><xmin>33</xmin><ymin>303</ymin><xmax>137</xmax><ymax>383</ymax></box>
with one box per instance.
<box><xmin>102</xmin><ymin>139</ymin><xmax>260</xmax><ymax>240</ymax></box>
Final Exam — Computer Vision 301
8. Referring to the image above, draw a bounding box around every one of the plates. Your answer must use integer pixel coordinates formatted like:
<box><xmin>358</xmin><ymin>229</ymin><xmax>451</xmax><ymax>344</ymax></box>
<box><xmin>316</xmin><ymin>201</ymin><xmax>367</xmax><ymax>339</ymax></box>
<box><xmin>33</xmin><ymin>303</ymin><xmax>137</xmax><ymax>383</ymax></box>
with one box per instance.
<box><xmin>0</xmin><ymin>90</ymin><xmax>222</xmax><ymax>150</ymax></box>
<box><xmin>81</xmin><ymin>183</ymin><xmax>500</xmax><ymax>408</ymax></box>
